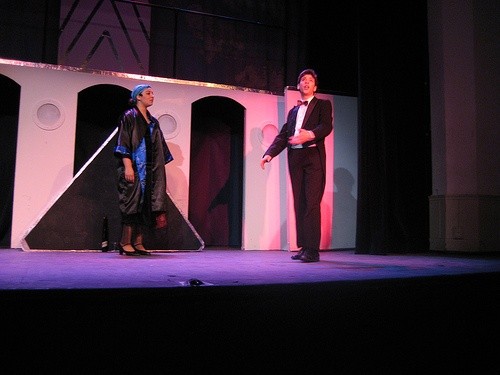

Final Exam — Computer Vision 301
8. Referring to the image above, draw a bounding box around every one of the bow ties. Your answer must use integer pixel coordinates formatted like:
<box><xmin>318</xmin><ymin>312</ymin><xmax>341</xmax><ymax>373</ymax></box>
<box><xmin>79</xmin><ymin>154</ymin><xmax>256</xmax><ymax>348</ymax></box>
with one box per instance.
<box><xmin>296</xmin><ymin>100</ymin><xmax>308</xmax><ymax>106</ymax></box>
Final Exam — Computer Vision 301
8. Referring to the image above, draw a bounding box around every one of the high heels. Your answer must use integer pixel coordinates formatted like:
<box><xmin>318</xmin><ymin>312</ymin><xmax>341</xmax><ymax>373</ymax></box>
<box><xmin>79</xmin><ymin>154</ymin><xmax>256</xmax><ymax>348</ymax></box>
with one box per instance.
<box><xmin>119</xmin><ymin>243</ymin><xmax>140</xmax><ymax>256</ymax></box>
<box><xmin>132</xmin><ymin>244</ymin><xmax>150</xmax><ymax>255</ymax></box>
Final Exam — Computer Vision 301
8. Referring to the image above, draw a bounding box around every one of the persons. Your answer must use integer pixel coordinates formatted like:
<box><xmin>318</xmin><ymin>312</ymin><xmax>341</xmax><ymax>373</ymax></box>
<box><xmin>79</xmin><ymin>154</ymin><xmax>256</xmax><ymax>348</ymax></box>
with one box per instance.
<box><xmin>113</xmin><ymin>84</ymin><xmax>173</xmax><ymax>256</ymax></box>
<box><xmin>259</xmin><ymin>69</ymin><xmax>333</xmax><ymax>263</ymax></box>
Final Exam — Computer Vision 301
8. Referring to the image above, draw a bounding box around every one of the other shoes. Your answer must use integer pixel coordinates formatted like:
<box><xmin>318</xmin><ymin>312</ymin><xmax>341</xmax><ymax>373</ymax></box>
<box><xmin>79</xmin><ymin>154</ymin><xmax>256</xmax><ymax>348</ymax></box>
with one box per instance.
<box><xmin>290</xmin><ymin>250</ymin><xmax>304</xmax><ymax>260</ymax></box>
<box><xmin>299</xmin><ymin>250</ymin><xmax>319</xmax><ymax>262</ymax></box>
